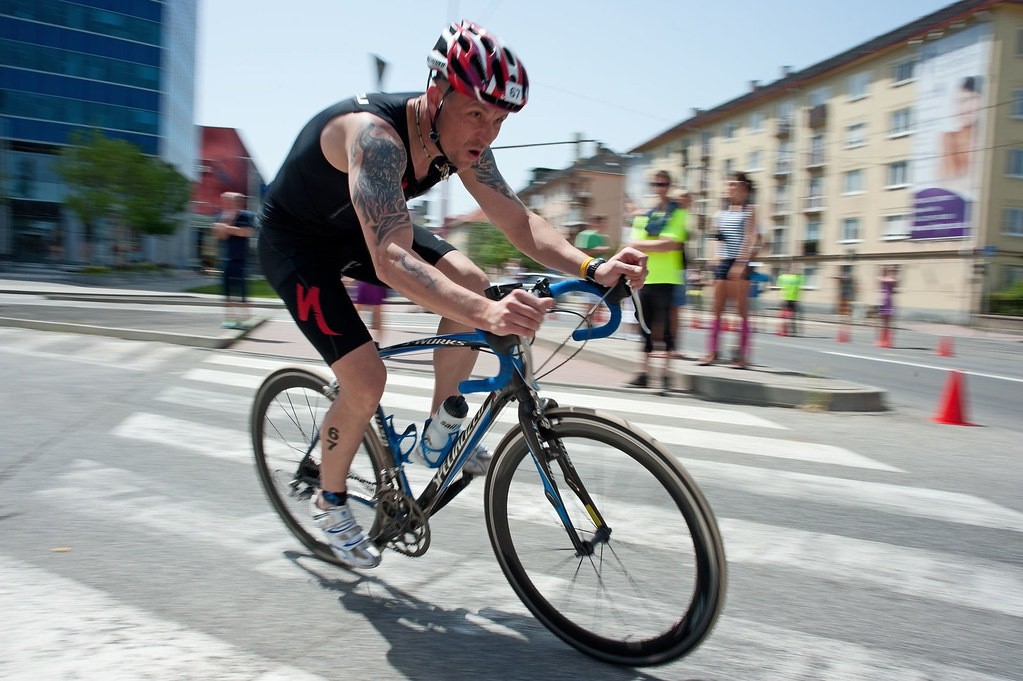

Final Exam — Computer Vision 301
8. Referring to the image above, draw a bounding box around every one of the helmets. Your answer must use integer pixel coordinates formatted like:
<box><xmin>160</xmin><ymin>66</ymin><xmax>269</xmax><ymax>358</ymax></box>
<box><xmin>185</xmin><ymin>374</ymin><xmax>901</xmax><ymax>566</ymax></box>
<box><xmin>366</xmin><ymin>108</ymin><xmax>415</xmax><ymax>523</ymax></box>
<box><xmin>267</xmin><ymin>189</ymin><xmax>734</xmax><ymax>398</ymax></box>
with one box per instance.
<box><xmin>427</xmin><ymin>18</ymin><xmax>529</xmax><ymax>113</ymax></box>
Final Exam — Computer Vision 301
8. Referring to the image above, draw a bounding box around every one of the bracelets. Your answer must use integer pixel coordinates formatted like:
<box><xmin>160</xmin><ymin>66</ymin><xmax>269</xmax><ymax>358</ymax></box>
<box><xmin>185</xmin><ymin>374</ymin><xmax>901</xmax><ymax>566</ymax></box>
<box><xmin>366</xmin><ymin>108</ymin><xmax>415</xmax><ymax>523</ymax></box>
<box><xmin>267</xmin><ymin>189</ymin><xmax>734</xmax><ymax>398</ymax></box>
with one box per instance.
<box><xmin>580</xmin><ymin>257</ymin><xmax>606</xmax><ymax>283</ymax></box>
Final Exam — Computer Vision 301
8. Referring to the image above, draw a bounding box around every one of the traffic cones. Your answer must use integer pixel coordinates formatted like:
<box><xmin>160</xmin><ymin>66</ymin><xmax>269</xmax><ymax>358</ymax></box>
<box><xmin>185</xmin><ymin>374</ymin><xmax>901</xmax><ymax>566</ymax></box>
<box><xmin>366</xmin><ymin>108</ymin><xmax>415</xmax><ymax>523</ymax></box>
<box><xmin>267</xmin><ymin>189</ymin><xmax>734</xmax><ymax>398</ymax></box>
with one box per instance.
<box><xmin>933</xmin><ymin>369</ymin><xmax>970</xmax><ymax>424</ymax></box>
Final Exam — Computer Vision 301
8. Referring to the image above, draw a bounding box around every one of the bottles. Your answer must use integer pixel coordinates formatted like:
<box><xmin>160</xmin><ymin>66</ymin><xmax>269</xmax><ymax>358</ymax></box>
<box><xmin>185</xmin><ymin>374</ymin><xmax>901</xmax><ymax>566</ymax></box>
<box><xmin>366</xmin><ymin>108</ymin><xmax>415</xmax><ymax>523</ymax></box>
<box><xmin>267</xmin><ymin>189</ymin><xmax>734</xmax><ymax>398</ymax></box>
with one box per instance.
<box><xmin>414</xmin><ymin>395</ymin><xmax>469</xmax><ymax>467</ymax></box>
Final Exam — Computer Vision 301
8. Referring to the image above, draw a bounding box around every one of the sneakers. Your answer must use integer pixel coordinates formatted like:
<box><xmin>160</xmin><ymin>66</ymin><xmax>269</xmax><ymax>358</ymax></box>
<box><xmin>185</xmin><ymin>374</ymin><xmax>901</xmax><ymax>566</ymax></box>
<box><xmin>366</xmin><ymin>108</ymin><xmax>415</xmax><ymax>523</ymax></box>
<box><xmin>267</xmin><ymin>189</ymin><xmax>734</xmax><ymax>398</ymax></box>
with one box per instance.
<box><xmin>297</xmin><ymin>489</ymin><xmax>382</xmax><ymax>569</ymax></box>
<box><xmin>414</xmin><ymin>428</ymin><xmax>492</xmax><ymax>475</ymax></box>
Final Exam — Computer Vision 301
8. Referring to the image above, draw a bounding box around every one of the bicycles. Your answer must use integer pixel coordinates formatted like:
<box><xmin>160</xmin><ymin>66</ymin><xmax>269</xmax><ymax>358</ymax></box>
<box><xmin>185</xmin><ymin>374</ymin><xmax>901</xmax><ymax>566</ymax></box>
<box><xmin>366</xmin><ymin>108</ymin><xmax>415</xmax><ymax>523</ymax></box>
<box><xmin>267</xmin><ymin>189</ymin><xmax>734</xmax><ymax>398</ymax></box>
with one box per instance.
<box><xmin>249</xmin><ymin>279</ymin><xmax>727</xmax><ymax>667</ymax></box>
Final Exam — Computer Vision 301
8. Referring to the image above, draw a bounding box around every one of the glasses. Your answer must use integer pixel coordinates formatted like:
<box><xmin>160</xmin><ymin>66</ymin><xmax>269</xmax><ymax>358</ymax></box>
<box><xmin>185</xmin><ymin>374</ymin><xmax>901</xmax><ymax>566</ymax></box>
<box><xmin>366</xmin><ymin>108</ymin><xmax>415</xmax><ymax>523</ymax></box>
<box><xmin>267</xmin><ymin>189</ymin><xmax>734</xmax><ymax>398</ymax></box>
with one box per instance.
<box><xmin>724</xmin><ymin>180</ymin><xmax>749</xmax><ymax>189</ymax></box>
<box><xmin>648</xmin><ymin>182</ymin><xmax>670</xmax><ymax>187</ymax></box>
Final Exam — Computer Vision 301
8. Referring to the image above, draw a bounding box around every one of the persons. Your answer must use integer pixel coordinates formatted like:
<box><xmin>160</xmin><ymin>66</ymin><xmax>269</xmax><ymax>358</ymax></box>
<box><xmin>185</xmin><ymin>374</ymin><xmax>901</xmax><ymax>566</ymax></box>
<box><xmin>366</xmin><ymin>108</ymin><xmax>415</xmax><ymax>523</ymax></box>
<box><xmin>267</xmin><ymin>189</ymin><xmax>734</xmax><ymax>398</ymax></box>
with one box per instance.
<box><xmin>697</xmin><ymin>173</ymin><xmax>759</xmax><ymax>370</ymax></box>
<box><xmin>259</xmin><ymin>20</ymin><xmax>649</xmax><ymax>569</ymax></box>
<box><xmin>574</xmin><ymin>213</ymin><xmax>617</xmax><ymax>324</ymax></box>
<box><xmin>632</xmin><ymin>169</ymin><xmax>691</xmax><ymax>393</ymax></box>
<box><xmin>211</xmin><ymin>191</ymin><xmax>257</xmax><ymax>327</ymax></box>
<box><xmin>939</xmin><ymin>76</ymin><xmax>982</xmax><ymax>179</ymax></box>
<box><xmin>778</xmin><ymin>259</ymin><xmax>801</xmax><ymax>335</ymax></box>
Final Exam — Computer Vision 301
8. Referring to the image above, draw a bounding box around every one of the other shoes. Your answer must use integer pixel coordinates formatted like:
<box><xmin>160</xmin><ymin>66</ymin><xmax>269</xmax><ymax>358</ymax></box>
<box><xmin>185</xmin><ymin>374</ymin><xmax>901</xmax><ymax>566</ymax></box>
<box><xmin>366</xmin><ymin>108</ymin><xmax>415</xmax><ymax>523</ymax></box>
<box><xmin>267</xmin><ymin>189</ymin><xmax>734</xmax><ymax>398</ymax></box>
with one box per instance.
<box><xmin>671</xmin><ymin>351</ymin><xmax>685</xmax><ymax>356</ymax></box>
<box><xmin>625</xmin><ymin>371</ymin><xmax>649</xmax><ymax>387</ymax></box>
<box><xmin>661</xmin><ymin>377</ymin><xmax>671</xmax><ymax>388</ymax></box>
<box><xmin>728</xmin><ymin>356</ymin><xmax>746</xmax><ymax>368</ymax></box>
<box><xmin>696</xmin><ymin>351</ymin><xmax>718</xmax><ymax>365</ymax></box>
